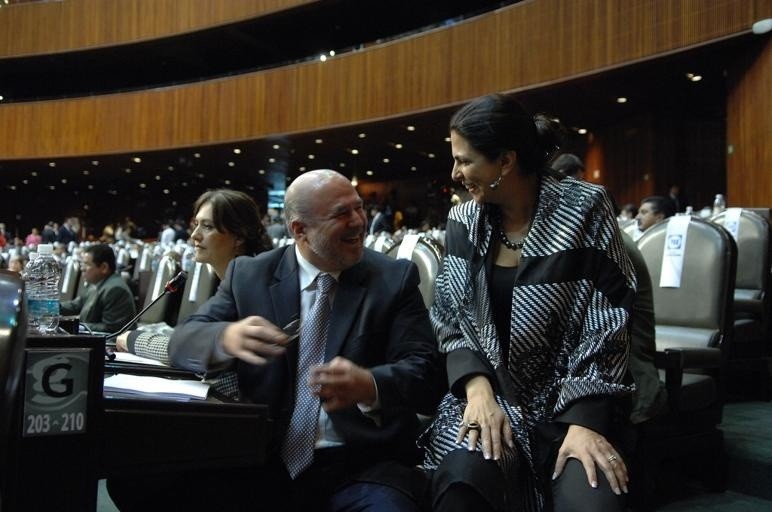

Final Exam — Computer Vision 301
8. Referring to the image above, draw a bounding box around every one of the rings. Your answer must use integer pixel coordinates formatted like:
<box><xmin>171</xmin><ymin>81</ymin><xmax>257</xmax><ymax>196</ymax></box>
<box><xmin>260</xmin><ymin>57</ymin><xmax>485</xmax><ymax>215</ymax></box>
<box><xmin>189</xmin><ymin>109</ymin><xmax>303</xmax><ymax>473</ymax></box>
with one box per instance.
<box><xmin>606</xmin><ymin>455</ymin><xmax>616</xmax><ymax>464</ymax></box>
<box><xmin>414</xmin><ymin>92</ymin><xmax>669</xmax><ymax>512</ymax></box>
<box><xmin>464</xmin><ymin>420</ymin><xmax>482</xmax><ymax>434</ymax></box>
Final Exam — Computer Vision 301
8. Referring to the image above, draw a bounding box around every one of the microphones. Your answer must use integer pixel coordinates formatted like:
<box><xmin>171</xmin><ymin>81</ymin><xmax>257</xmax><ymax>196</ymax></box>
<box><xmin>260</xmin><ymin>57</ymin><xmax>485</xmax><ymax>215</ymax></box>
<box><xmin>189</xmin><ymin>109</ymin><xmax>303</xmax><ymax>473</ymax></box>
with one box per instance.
<box><xmin>103</xmin><ymin>271</ymin><xmax>189</xmax><ymax>340</ymax></box>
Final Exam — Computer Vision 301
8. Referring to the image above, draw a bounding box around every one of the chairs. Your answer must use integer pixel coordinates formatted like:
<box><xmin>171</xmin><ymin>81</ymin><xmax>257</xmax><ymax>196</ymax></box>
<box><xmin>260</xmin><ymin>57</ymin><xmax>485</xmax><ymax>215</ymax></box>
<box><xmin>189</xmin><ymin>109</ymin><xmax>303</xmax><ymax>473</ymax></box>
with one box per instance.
<box><xmin>707</xmin><ymin>210</ymin><xmax>769</xmax><ymax>327</ymax></box>
<box><xmin>384</xmin><ymin>237</ymin><xmax>440</xmax><ymax>312</ymax></box>
<box><xmin>113</xmin><ymin>242</ymin><xmax>194</xmax><ymax>270</ymax></box>
<box><xmin>0</xmin><ymin>274</ymin><xmax>30</xmax><ymax>422</ymax></box>
<box><xmin>178</xmin><ymin>261</ymin><xmax>215</xmax><ymax>329</ymax></box>
<box><xmin>139</xmin><ymin>257</ymin><xmax>179</xmax><ymax>328</ymax></box>
<box><xmin>635</xmin><ymin>216</ymin><xmax>738</xmax><ymax>467</ymax></box>
<box><xmin>58</xmin><ymin>259</ymin><xmax>80</xmax><ymax>300</ymax></box>
<box><xmin>1</xmin><ymin>241</ymin><xmax>96</xmax><ymax>267</ymax></box>
<box><xmin>364</xmin><ymin>228</ymin><xmax>445</xmax><ymax>256</ymax></box>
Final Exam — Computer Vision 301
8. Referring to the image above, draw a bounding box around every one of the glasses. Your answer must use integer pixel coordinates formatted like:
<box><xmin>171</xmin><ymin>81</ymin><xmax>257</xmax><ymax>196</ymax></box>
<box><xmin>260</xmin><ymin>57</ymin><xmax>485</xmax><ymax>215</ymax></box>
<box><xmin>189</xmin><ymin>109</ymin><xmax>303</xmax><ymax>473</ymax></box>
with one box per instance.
<box><xmin>267</xmin><ymin>311</ymin><xmax>310</xmax><ymax>345</ymax></box>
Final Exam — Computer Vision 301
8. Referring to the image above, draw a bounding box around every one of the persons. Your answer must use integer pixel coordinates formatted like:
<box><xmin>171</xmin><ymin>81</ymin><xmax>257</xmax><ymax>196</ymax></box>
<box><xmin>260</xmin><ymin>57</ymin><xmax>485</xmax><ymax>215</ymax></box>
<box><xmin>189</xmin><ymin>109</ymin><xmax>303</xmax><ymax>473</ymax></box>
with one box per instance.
<box><xmin>58</xmin><ymin>244</ymin><xmax>137</xmax><ymax>334</ymax></box>
<box><xmin>634</xmin><ymin>194</ymin><xmax>677</xmax><ymax>231</ymax></box>
<box><xmin>41</xmin><ymin>220</ymin><xmax>59</xmax><ymax>244</ymax></box>
<box><xmin>620</xmin><ymin>204</ymin><xmax>637</xmax><ymax>220</ymax></box>
<box><xmin>366</xmin><ymin>205</ymin><xmax>390</xmax><ymax>237</ymax></box>
<box><xmin>161</xmin><ymin>220</ymin><xmax>175</xmax><ymax>245</ymax></box>
<box><xmin>57</xmin><ymin>217</ymin><xmax>79</xmax><ymax>244</ymax></box>
<box><xmin>167</xmin><ymin>168</ymin><xmax>447</xmax><ymax>511</ymax></box>
<box><xmin>26</xmin><ymin>228</ymin><xmax>41</xmax><ymax>246</ymax></box>
<box><xmin>172</xmin><ymin>218</ymin><xmax>191</xmax><ymax>243</ymax></box>
<box><xmin>105</xmin><ymin>189</ymin><xmax>273</xmax><ymax>512</ymax></box>
<box><xmin>550</xmin><ymin>154</ymin><xmax>584</xmax><ymax>181</ymax></box>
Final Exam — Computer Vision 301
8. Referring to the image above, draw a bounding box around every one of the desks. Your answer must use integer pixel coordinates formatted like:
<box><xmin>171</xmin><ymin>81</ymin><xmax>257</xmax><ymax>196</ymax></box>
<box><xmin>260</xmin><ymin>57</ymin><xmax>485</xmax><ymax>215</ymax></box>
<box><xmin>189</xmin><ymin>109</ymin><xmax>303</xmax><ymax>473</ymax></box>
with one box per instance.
<box><xmin>24</xmin><ymin>323</ymin><xmax>270</xmax><ymax>512</ymax></box>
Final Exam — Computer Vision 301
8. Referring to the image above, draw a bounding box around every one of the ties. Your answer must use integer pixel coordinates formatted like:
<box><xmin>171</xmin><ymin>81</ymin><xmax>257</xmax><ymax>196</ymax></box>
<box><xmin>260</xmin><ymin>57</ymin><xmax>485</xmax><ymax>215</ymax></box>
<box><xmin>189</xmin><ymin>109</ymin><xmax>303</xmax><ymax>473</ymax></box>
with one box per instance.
<box><xmin>278</xmin><ymin>273</ymin><xmax>337</xmax><ymax>480</ymax></box>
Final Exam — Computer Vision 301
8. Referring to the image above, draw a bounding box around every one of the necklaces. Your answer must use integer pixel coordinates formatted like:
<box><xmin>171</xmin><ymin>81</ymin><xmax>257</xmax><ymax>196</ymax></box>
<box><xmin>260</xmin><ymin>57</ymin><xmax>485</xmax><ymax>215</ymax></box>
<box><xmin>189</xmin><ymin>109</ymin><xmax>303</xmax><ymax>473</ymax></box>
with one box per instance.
<box><xmin>495</xmin><ymin>209</ymin><xmax>530</xmax><ymax>251</ymax></box>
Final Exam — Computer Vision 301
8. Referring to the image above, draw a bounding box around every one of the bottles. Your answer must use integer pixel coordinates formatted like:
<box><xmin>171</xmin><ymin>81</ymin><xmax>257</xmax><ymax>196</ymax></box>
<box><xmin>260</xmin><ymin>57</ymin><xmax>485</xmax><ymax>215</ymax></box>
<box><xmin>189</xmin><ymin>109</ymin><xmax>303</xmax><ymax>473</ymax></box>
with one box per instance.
<box><xmin>713</xmin><ymin>194</ymin><xmax>725</xmax><ymax>217</ymax></box>
<box><xmin>30</xmin><ymin>243</ymin><xmax>61</xmax><ymax>336</ymax></box>
<box><xmin>21</xmin><ymin>252</ymin><xmax>37</xmax><ymax>305</ymax></box>
<box><xmin>685</xmin><ymin>206</ymin><xmax>693</xmax><ymax>217</ymax></box>
